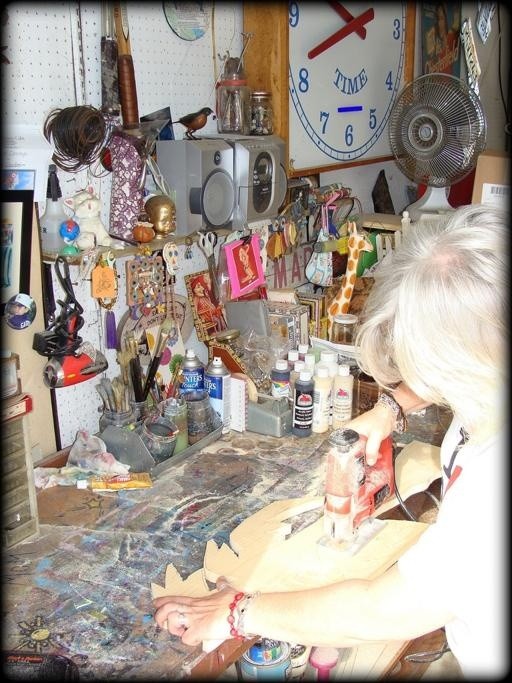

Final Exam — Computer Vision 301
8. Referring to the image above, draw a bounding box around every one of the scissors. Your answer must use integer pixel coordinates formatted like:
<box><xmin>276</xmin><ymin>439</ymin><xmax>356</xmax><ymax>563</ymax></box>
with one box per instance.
<box><xmin>197</xmin><ymin>231</ymin><xmax>219</xmax><ymax>300</ymax></box>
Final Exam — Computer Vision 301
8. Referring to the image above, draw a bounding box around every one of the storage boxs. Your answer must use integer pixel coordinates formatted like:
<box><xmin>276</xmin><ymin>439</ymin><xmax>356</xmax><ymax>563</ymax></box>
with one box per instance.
<box><xmin>471</xmin><ymin>149</ymin><xmax>512</xmax><ymax>205</ymax></box>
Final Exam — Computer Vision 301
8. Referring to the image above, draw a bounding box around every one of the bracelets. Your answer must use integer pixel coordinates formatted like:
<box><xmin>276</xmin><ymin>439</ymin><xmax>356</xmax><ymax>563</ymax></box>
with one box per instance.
<box><xmin>379</xmin><ymin>388</ymin><xmax>411</xmax><ymax>436</ymax></box>
<box><xmin>226</xmin><ymin>589</ymin><xmax>262</xmax><ymax>643</ymax></box>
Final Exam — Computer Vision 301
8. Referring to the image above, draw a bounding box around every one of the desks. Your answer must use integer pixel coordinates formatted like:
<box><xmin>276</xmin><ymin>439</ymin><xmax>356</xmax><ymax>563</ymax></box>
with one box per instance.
<box><xmin>0</xmin><ymin>427</ymin><xmax>420</xmax><ymax>683</ymax></box>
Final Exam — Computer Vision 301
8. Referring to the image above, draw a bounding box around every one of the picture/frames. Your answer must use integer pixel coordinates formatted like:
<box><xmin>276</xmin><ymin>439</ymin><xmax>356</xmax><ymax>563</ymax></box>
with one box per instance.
<box><xmin>0</xmin><ymin>190</ymin><xmax>34</xmax><ymax>310</ymax></box>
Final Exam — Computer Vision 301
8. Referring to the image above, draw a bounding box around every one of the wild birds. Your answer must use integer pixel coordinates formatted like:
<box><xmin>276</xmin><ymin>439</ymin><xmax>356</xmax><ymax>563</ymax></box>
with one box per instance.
<box><xmin>168</xmin><ymin>108</ymin><xmax>215</xmax><ymax>140</ymax></box>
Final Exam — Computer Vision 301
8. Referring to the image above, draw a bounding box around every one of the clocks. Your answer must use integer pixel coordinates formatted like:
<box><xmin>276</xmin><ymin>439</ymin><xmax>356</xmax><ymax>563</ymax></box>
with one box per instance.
<box><xmin>289</xmin><ymin>1</ymin><xmax>409</xmax><ymax>161</ymax></box>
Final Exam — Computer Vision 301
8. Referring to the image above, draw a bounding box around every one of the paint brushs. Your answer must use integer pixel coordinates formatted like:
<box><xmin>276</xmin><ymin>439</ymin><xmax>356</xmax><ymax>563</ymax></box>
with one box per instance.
<box><xmin>95</xmin><ymin>329</ymin><xmax>169</xmax><ymax>413</ymax></box>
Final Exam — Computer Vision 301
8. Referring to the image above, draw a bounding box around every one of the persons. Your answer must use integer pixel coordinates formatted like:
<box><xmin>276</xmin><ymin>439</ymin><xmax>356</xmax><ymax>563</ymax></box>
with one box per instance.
<box><xmin>424</xmin><ymin>0</ymin><xmax>457</xmax><ymax>88</ymax></box>
<box><xmin>7</xmin><ymin>292</ymin><xmax>33</xmax><ymax>328</ymax></box>
<box><xmin>144</xmin><ymin>191</ymin><xmax>178</xmax><ymax>239</ymax></box>
<box><xmin>148</xmin><ymin>197</ymin><xmax>507</xmax><ymax>679</ymax></box>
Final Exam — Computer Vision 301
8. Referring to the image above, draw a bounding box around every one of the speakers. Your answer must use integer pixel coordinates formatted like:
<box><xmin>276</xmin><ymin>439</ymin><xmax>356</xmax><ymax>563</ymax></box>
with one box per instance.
<box><xmin>155</xmin><ymin>139</ymin><xmax>234</xmax><ymax>238</ymax></box>
<box><xmin>183</xmin><ymin>136</ymin><xmax>288</xmax><ymax>224</ymax></box>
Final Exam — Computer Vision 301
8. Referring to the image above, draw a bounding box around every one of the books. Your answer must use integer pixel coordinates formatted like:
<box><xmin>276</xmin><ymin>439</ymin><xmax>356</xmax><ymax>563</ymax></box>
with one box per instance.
<box><xmin>261</xmin><ymin>284</ymin><xmax>330</xmax><ymax>351</ymax></box>
<box><xmin>230</xmin><ymin>374</ymin><xmax>249</xmax><ymax>435</ymax></box>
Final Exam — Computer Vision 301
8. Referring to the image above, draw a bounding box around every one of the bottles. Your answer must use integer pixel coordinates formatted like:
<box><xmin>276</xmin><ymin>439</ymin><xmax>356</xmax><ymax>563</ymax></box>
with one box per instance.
<box><xmin>330</xmin><ymin>312</ymin><xmax>359</xmax><ymax>342</ymax></box>
<box><xmin>215</xmin><ymin>80</ymin><xmax>276</xmax><ymax>136</ymax></box>
<box><xmin>98</xmin><ymin>349</ymin><xmax>230</xmax><ymax>464</ymax></box>
<box><xmin>270</xmin><ymin>343</ymin><xmax>354</xmax><ymax>437</ymax></box>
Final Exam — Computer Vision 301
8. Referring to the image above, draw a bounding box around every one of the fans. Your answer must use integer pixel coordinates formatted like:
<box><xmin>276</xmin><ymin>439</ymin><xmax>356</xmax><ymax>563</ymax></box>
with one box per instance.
<box><xmin>388</xmin><ymin>72</ymin><xmax>487</xmax><ymax>223</ymax></box>
